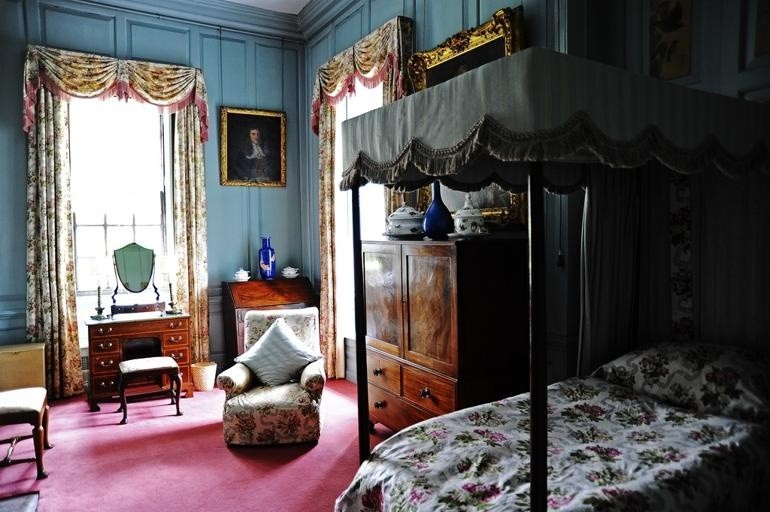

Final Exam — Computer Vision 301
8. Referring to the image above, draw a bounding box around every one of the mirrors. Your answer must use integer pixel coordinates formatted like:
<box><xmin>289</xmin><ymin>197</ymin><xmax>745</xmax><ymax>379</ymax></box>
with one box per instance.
<box><xmin>114</xmin><ymin>243</ymin><xmax>154</xmax><ymax>293</ymax></box>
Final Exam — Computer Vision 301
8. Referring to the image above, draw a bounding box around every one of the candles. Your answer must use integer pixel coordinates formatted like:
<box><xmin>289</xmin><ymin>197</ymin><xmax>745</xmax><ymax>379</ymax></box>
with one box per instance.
<box><xmin>169</xmin><ymin>283</ymin><xmax>172</xmax><ymax>301</ymax></box>
<box><xmin>98</xmin><ymin>287</ymin><xmax>100</xmax><ymax>307</ymax></box>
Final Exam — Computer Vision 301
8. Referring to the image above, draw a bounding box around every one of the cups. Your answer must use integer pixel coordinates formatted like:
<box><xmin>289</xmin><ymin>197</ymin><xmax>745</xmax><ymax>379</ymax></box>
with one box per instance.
<box><xmin>451</xmin><ymin>193</ymin><xmax>485</xmax><ymax>234</ymax></box>
<box><xmin>233</xmin><ymin>267</ymin><xmax>251</xmax><ymax>278</ymax></box>
<box><xmin>281</xmin><ymin>266</ymin><xmax>297</xmax><ymax>275</ymax></box>
<box><xmin>385</xmin><ymin>202</ymin><xmax>426</xmax><ymax>235</ymax></box>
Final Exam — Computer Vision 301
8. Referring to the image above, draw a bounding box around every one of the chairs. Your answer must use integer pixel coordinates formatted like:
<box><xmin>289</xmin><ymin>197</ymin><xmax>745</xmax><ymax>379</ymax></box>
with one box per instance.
<box><xmin>216</xmin><ymin>306</ymin><xmax>326</xmax><ymax>446</ymax></box>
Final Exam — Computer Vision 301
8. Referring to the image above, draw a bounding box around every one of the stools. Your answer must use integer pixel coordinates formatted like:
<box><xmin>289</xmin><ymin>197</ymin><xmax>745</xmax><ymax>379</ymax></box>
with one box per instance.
<box><xmin>117</xmin><ymin>356</ymin><xmax>183</xmax><ymax>424</ymax></box>
<box><xmin>0</xmin><ymin>387</ymin><xmax>54</xmax><ymax>480</ymax></box>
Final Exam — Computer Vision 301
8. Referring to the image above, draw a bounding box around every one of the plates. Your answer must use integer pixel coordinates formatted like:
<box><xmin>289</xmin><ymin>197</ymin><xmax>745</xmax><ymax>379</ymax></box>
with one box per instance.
<box><xmin>380</xmin><ymin>232</ymin><xmax>427</xmax><ymax>241</ymax></box>
<box><xmin>445</xmin><ymin>232</ymin><xmax>493</xmax><ymax>242</ymax></box>
<box><xmin>281</xmin><ymin>273</ymin><xmax>299</xmax><ymax>279</ymax></box>
<box><xmin>232</xmin><ymin>276</ymin><xmax>251</xmax><ymax>282</ymax></box>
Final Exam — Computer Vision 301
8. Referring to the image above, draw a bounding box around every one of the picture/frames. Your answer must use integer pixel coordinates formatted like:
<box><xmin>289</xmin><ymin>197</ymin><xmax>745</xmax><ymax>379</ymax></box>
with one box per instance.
<box><xmin>407</xmin><ymin>5</ymin><xmax>528</xmax><ymax>227</ymax></box>
<box><xmin>220</xmin><ymin>106</ymin><xmax>287</xmax><ymax>188</ymax></box>
<box><xmin>649</xmin><ymin>0</ymin><xmax>693</xmax><ymax>79</ymax></box>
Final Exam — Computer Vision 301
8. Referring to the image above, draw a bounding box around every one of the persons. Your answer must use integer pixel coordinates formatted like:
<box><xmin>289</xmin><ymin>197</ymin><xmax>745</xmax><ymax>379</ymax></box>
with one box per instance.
<box><xmin>233</xmin><ymin>124</ymin><xmax>275</xmax><ymax>180</ymax></box>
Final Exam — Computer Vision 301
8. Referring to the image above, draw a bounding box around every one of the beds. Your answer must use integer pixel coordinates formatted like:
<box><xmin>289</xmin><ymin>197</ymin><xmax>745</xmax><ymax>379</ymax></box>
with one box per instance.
<box><xmin>340</xmin><ymin>45</ymin><xmax>770</xmax><ymax>512</ymax></box>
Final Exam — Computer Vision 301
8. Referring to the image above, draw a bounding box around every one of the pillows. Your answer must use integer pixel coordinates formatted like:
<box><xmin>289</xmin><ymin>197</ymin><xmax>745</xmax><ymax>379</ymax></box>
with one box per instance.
<box><xmin>590</xmin><ymin>341</ymin><xmax>770</xmax><ymax>422</ymax></box>
<box><xmin>233</xmin><ymin>317</ymin><xmax>322</xmax><ymax>387</ymax></box>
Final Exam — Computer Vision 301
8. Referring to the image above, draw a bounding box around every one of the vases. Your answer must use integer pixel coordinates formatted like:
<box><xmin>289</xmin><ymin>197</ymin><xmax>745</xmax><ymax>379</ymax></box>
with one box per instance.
<box><xmin>259</xmin><ymin>236</ymin><xmax>277</xmax><ymax>280</ymax></box>
<box><xmin>422</xmin><ymin>180</ymin><xmax>454</xmax><ymax>241</ymax></box>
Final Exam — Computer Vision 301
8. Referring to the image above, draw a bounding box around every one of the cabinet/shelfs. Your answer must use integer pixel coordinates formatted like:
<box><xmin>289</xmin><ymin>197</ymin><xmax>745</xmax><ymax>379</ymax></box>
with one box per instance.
<box><xmin>221</xmin><ymin>277</ymin><xmax>319</xmax><ymax>371</ymax></box>
<box><xmin>0</xmin><ymin>341</ymin><xmax>47</xmax><ymax>391</ymax></box>
<box><xmin>362</xmin><ymin>237</ymin><xmax>529</xmax><ymax>434</ymax></box>
<box><xmin>85</xmin><ymin>309</ymin><xmax>194</xmax><ymax>412</ymax></box>
